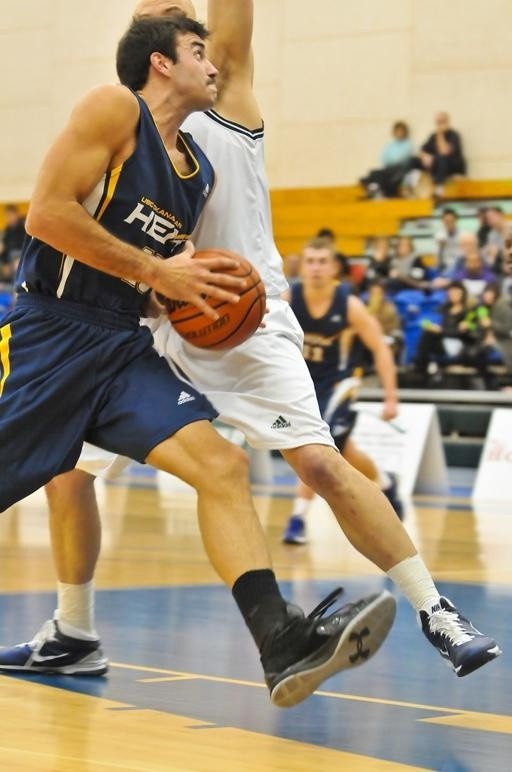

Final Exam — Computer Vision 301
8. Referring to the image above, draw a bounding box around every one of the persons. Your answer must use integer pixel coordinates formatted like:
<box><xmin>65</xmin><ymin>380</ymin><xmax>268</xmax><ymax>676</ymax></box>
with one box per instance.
<box><xmin>284</xmin><ymin>240</ymin><xmax>404</xmax><ymax>545</ymax></box>
<box><xmin>0</xmin><ymin>204</ymin><xmax>30</xmax><ymax>265</ymax></box>
<box><xmin>0</xmin><ymin>0</ymin><xmax>503</xmax><ymax>678</ymax></box>
<box><xmin>365</xmin><ymin>112</ymin><xmax>465</xmax><ymax>200</ymax></box>
<box><xmin>285</xmin><ymin>206</ymin><xmax>512</xmax><ymax>395</ymax></box>
<box><xmin>1</xmin><ymin>15</ymin><xmax>398</xmax><ymax>706</ymax></box>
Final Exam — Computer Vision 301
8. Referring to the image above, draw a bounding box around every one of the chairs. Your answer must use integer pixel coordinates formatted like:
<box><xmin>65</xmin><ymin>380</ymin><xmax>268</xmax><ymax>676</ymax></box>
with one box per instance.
<box><xmin>321</xmin><ymin>195</ymin><xmax>512</xmax><ymax>468</ymax></box>
<box><xmin>1</xmin><ymin>232</ymin><xmax>27</xmax><ymax>317</ymax></box>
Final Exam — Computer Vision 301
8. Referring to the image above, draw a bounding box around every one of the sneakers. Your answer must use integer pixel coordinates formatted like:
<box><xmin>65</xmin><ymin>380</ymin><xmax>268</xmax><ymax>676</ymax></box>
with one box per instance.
<box><xmin>415</xmin><ymin>594</ymin><xmax>502</xmax><ymax>676</ymax></box>
<box><xmin>260</xmin><ymin>588</ymin><xmax>398</xmax><ymax>708</ymax></box>
<box><xmin>1</xmin><ymin>617</ymin><xmax>108</xmax><ymax>676</ymax></box>
<box><xmin>283</xmin><ymin>513</ymin><xmax>308</xmax><ymax>546</ymax></box>
<box><xmin>380</xmin><ymin>469</ymin><xmax>404</xmax><ymax>522</ymax></box>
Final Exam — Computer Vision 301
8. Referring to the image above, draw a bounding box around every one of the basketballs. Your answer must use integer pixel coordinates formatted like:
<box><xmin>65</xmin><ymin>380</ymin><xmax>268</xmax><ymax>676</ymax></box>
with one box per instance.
<box><xmin>166</xmin><ymin>248</ymin><xmax>265</xmax><ymax>351</ymax></box>
